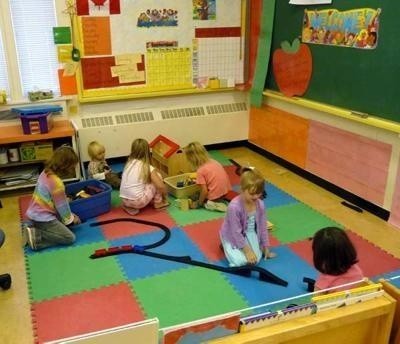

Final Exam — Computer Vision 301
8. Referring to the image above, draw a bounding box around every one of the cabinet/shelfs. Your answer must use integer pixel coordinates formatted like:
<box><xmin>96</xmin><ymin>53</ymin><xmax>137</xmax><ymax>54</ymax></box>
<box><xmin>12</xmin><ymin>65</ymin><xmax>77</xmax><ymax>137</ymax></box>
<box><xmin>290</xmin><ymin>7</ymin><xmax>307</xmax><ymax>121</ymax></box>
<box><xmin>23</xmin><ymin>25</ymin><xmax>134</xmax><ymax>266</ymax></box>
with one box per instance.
<box><xmin>0</xmin><ymin>119</ymin><xmax>81</xmax><ymax>192</ymax></box>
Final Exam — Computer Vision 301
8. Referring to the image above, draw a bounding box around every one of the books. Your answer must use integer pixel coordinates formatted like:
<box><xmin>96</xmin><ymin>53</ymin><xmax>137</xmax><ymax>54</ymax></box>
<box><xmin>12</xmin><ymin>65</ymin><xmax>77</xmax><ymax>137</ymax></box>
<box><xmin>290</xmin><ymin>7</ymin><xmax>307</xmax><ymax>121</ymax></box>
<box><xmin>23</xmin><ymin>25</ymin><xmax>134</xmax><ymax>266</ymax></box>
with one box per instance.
<box><xmin>240</xmin><ymin>303</ymin><xmax>317</xmax><ymax>334</ymax></box>
<box><xmin>163</xmin><ymin>312</ymin><xmax>240</xmax><ymax>344</ymax></box>
<box><xmin>312</xmin><ymin>282</ymin><xmax>384</xmax><ymax>311</ymax></box>
<box><xmin>1</xmin><ymin>167</ymin><xmax>41</xmax><ymax>186</ymax></box>
<box><xmin>42</xmin><ymin>317</ymin><xmax>159</xmax><ymax>344</ymax></box>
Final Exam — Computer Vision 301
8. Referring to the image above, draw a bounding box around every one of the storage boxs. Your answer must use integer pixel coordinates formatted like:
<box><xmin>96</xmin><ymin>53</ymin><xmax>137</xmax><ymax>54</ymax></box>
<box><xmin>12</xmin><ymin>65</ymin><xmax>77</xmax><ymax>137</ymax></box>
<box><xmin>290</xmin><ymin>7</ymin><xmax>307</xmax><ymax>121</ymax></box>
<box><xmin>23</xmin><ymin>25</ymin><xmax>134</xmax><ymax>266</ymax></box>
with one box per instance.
<box><xmin>65</xmin><ymin>178</ymin><xmax>112</xmax><ymax>223</ymax></box>
<box><xmin>18</xmin><ymin>107</ymin><xmax>54</xmax><ymax>135</ymax></box>
<box><xmin>163</xmin><ymin>173</ymin><xmax>201</xmax><ymax>199</ymax></box>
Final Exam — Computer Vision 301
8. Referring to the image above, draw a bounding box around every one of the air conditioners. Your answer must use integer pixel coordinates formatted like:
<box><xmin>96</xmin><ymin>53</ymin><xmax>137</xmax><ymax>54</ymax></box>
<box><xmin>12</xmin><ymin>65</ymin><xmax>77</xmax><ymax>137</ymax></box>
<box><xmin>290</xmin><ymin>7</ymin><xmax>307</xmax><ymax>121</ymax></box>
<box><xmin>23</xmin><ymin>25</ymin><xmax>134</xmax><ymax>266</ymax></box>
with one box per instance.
<box><xmin>78</xmin><ymin>102</ymin><xmax>249</xmax><ymax>162</ymax></box>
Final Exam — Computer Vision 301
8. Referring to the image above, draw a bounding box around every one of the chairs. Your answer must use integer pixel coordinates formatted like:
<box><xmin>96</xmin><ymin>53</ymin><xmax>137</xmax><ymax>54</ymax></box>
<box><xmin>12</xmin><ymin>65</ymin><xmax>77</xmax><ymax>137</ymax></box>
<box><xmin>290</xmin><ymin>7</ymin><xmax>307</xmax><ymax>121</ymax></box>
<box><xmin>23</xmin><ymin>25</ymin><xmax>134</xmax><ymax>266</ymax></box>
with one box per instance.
<box><xmin>0</xmin><ymin>200</ymin><xmax>11</xmax><ymax>290</ymax></box>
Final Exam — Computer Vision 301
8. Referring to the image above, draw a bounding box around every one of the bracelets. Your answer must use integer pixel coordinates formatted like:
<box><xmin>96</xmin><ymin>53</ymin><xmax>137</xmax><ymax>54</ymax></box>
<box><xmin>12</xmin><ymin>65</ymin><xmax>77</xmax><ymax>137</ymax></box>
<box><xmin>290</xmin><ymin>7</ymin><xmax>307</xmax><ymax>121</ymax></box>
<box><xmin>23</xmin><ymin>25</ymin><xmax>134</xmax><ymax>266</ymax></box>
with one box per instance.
<box><xmin>197</xmin><ymin>202</ymin><xmax>203</xmax><ymax>207</ymax></box>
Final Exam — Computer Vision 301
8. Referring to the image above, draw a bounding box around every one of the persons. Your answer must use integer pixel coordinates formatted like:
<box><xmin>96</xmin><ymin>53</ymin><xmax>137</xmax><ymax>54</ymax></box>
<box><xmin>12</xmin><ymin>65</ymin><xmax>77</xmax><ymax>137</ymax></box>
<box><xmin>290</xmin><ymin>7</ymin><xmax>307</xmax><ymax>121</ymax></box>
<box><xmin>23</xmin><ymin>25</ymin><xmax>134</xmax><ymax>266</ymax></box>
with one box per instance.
<box><xmin>87</xmin><ymin>141</ymin><xmax>121</xmax><ymax>188</ymax></box>
<box><xmin>119</xmin><ymin>139</ymin><xmax>170</xmax><ymax>215</ymax></box>
<box><xmin>25</xmin><ymin>146</ymin><xmax>80</xmax><ymax>251</ymax></box>
<box><xmin>220</xmin><ymin>171</ymin><xmax>276</xmax><ymax>268</ymax></box>
<box><xmin>185</xmin><ymin>142</ymin><xmax>232</xmax><ymax>208</ymax></box>
<box><xmin>312</xmin><ymin>226</ymin><xmax>363</xmax><ymax>291</ymax></box>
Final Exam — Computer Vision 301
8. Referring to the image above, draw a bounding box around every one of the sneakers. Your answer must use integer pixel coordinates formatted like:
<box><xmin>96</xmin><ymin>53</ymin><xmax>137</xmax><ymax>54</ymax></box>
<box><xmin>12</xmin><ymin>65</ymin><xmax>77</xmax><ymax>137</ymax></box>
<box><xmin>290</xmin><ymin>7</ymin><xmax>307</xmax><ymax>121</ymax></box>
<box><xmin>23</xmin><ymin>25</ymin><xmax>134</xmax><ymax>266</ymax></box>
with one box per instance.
<box><xmin>205</xmin><ymin>199</ymin><xmax>227</xmax><ymax>212</ymax></box>
<box><xmin>154</xmin><ymin>200</ymin><xmax>169</xmax><ymax>209</ymax></box>
<box><xmin>23</xmin><ymin>223</ymin><xmax>38</xmax><ymax>251</ymax></box>
<box><xmin>120</xmin><ymin>202</ymin><xmax>140</xmax><ymax>215</ymax></box>
